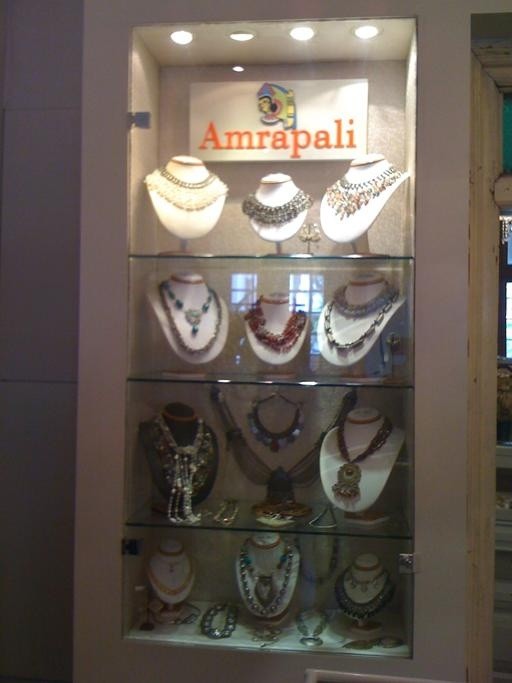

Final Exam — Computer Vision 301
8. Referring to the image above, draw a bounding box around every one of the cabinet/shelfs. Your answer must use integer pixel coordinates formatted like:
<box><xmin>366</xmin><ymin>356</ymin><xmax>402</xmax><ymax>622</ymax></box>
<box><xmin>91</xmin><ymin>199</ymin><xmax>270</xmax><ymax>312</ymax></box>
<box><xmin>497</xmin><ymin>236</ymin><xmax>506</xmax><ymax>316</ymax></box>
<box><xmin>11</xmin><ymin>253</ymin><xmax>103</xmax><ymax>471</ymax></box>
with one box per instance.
<box><xmin>125</xmin><ymin>252</ymin><xmax>414</xmax><ymax>658</ymax></box>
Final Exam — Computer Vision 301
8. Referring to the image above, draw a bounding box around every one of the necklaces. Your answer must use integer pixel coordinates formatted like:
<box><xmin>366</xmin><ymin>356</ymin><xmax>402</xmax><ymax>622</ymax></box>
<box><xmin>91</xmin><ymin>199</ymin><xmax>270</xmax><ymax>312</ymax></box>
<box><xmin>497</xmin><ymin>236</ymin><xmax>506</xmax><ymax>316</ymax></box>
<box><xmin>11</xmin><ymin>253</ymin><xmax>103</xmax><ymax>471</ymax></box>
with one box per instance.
<box><xmin>323</xmin><ymin>284</ymin><xmax>400</xmax><ymax>352</ymax></box>
<box><xmin>201</xmin><ymin>602</ymin><xmax>236</xmax><ymax>639</ymax></box>
<box><xmin>146</xmin><ymin>556</ymin><xmax>193</xmax><ymax>596</ymax></box>
<box><xmin>157</xmin><ymin>281</ymin><xmax>223</xmax><ymax>355</ymax></box>
<box><xmin>247</xmin><ymin>405</ymin><xmax>304</xmax><ymax>453</ymax></box>
<box><xmin>332</xmin><ymin>416</ymin><xmax>393</xmax><ymax>498</ymax></box>
<box><xmin>241</xmin><ymin>188</ymin><xmax>314</xmax><ymax>224</ymax></box>
<box><xmin>145</xmin><ymin>413</ymin><xmax>212</xmax><ymax>522</ymax></box>
<box><xmin>145</xmin><ymin>165</ymin><xmax>228</xmax><ymax>211</ymax></box>
<box><xmin>327</xmin><ymin>165</ymin><xmax>402</xmax><ymax>220</ymax></box>
<box><xmin>341</xmin><ymin>636</ymin><xmax>403</xmax><ymax>652</ymax></box>
<box><xmin>334</xmin><ymin>564</ymin><xmax>397</xmax><ymax>619</ymax></box>
<box><xmin>244</xmin><ymin>301</ymin><xmax>307</xmax><ymax>353</ymax></box>
<box><xmin>295</xmin><ymin>610</ymin><xmax>328</xmax><ymax>646</ymax></box>
<box><xmin>295</xmin><ymin>537</ymin><xmax>341</xmax><ymax>583</ymax></box>
<box><xmin>239</xmin><ymin>537</ymin><xmax>295</xmax><ymax>615</ymax></box>
